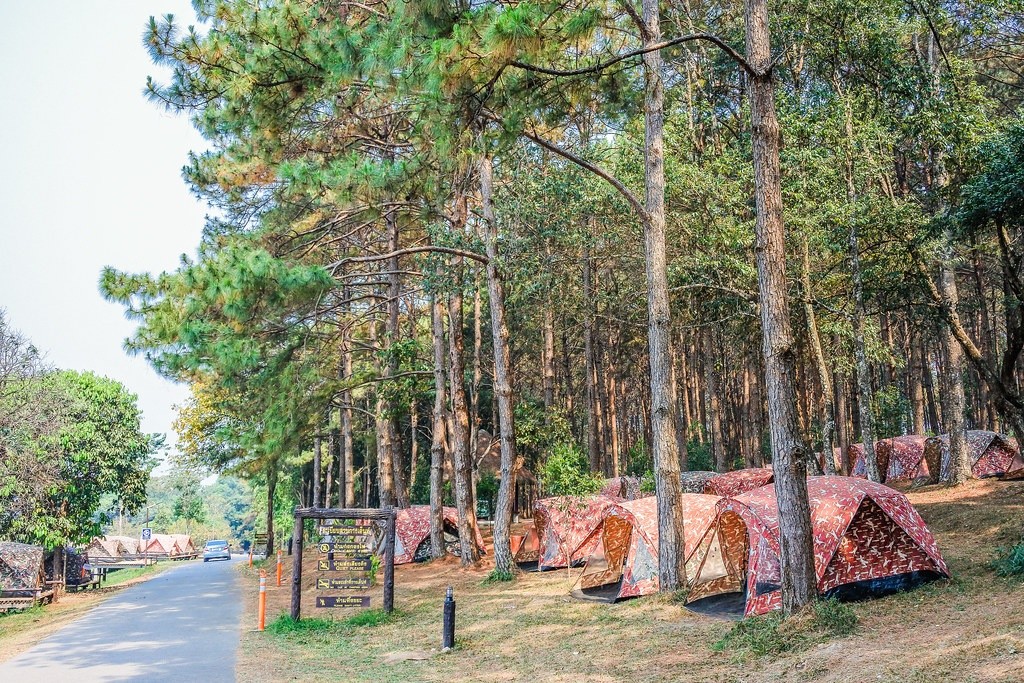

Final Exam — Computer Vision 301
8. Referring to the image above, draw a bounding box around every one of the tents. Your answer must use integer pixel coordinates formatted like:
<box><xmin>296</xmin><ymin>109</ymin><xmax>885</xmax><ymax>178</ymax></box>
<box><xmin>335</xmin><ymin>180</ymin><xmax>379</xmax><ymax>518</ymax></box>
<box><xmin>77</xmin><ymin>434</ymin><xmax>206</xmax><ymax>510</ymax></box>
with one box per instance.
<box><xmin>591</xmin><ymin>475</ymin><xmax>622</xmax><ymax>499</ymax></box>
<box><xmin>679</xmin><ymin>469</ymin><xmax>719</xmax><ymax>494</ymax></box>
<box><xmin>507</xmin><ymin>495</ymin><xmax>618</xmax><ymax>572</ymax></box>
<box><xmin>0</xmin><ymin>540</ymin><xmax>47</xmax><ymax>598</ymax></box>
<box><xmin>813</xmin><ymin>446</ymin><xmax>844</xmax><ymax>477</ymax></box>
<box><xmin>874</xmin><ymin>434</ymin><xmax>931</xmax><ymax>485</ymax></box>
<box><xmin>907</xmin><ymin>429</ymin><xmax>1023</xmax><ymax>488</ymax></box>
<box><xmin>847</xmin><ymin>442</ymin><xmax>870</xmax><ymax>480</ymax></box>
<box><xmin>44</xmin><ymin>546</ymin><xmax>92</xmax><ymax>584</ymax></box>
<box><xmin>616</xmin><ymin>475</ymin><xmax>656</xmax><ymax>500</ymax></box>
<box><xmin>350</xmin><ymin>504</ymin><xmax>489</xmax><ymax>566</ymax></box>
<box><xmin>559</xmin><ymin>493</ymin><xmax>725</xmax><ymax>605</ymax></box>
<box><xmin>705</xmin><ymin>467</ymin><xmax>774</xmax><ymax>497</ymax></box>
<box><xmin>682</xmin><ymin>475</ymin><xmax>951</xmax><ymax>620</ymax></box>
<box><xmin>83</xmin><ymin>533</ymin><xmax>196</xmax><ymax>561</ymax></box>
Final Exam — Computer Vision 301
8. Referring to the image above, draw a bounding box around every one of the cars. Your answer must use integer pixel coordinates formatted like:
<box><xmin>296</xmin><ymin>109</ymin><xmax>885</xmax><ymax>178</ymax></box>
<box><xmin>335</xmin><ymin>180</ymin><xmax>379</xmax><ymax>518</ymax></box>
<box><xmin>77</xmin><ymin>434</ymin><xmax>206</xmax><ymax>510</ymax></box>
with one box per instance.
<box><xmin>202</xmin><ymin>540</ymin><xmax>232</xmax><ymax>561</ymax></box>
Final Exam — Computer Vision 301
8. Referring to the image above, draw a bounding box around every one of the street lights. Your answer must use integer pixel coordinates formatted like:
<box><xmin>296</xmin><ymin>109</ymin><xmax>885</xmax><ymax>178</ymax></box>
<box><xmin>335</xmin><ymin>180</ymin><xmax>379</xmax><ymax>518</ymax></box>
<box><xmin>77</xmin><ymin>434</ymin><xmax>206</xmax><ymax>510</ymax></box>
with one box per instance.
<box><xmin>143</xmin><ymin>500</ymin><xmax>164</xmax><ymax>567</ymax></box>
